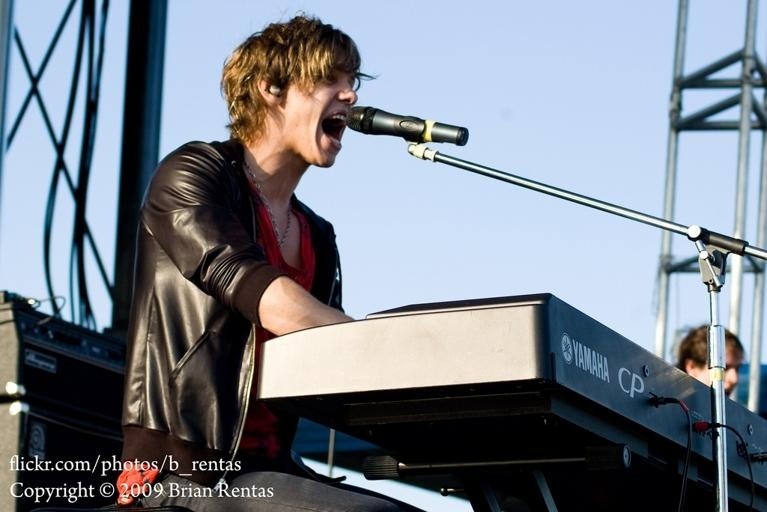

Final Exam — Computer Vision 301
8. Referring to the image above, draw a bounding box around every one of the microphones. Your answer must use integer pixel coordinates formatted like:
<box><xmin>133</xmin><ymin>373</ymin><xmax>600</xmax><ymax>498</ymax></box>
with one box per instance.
<box><xmin>344</xmin><ymin>106</ymin><xmax>469</xmax><ymax>147</ymax></box>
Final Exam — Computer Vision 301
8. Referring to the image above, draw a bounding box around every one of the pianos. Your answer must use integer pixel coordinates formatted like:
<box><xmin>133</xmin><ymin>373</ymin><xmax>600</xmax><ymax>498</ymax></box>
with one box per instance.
<box><xmin>254</xmin><ymin>293</ymin><xmax>766</xmax><ymax>510</ymax></box>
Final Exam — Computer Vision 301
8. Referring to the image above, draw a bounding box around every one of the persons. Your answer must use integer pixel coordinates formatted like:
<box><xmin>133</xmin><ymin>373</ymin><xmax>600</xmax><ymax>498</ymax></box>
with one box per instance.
<box><xmin>674</xmin><ymin>322</ymin><xmax>746</xmax><ymax>400</ymax></box>
<box><xmin>118</xmin><ymin>8</ymin><xmax>407</xmax><ymax>512</ymax></box>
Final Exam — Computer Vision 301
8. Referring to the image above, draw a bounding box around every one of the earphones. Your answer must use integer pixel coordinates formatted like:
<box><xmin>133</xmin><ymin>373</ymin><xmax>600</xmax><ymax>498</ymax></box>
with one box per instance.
<box><xmin>269</xmin><ymin>84</ymin><xmax>281</xmax><ymax>95</ymax></box>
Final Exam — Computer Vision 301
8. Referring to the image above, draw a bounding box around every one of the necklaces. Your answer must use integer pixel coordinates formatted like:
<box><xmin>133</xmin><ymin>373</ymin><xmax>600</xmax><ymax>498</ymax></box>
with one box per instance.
<box><xmin>243</xmin><ymin>158</ymin><xmax>293</xmax><ymax>251</ymax></box>
